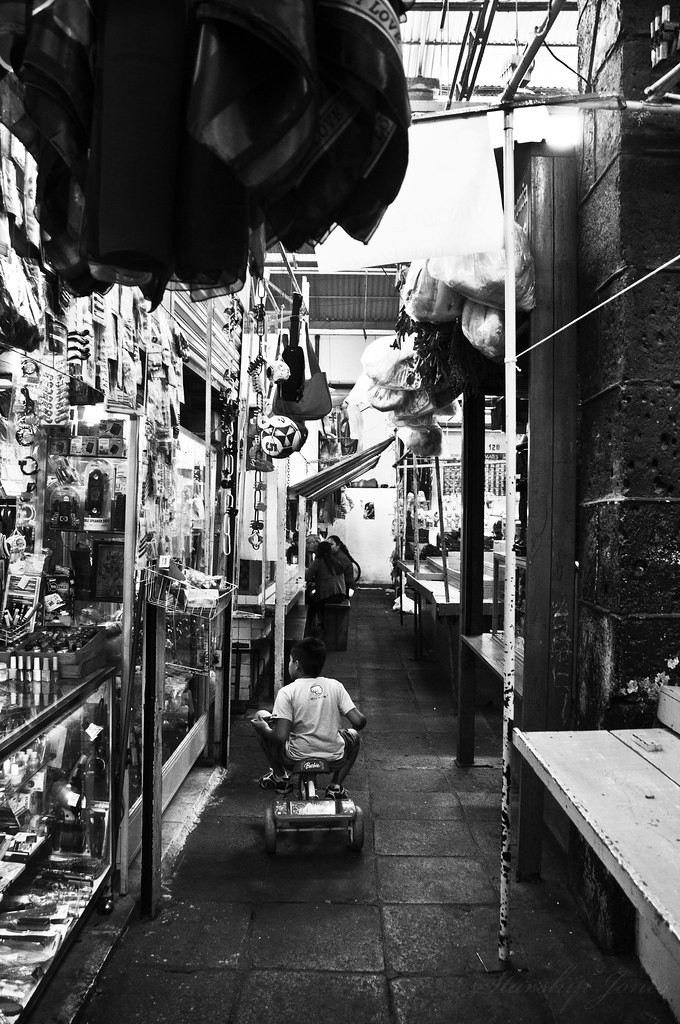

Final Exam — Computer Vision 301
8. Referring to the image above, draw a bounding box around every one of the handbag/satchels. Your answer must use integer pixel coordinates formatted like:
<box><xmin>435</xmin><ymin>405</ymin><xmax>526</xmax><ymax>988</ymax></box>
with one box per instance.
<box><xmin>271</xmin><ymin>372</ymin><xmax>333</xmax><ymax>421</ymax></box>
<box><xmin>362</xmin><ymin>216</ymin><xmax>536</xmax><ymax>449</ymax></box>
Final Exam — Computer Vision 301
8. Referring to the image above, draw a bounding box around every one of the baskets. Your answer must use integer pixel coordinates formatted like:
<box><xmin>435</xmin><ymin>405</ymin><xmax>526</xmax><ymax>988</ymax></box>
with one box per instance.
<box><xmin>144</xmin><ymin>561</ymin><xmax>240</xmax><ymax>621</ymax></box>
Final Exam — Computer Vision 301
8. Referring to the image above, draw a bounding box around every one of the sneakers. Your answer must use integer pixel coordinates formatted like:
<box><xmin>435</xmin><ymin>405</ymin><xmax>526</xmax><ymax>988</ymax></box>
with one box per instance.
<box><xmin>258</xmin><ymin>766</ymin><xmax>294</xmax><ymax>795</ymax></box>
<box><xmin>324</xmin><ymin>784</ymin><xmax>350</xmax><ymax>801</ymax></box>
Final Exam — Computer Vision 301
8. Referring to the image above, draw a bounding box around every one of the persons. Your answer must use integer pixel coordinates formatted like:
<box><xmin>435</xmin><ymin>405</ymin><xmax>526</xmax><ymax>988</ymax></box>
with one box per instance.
<box><xmin>251</xmin><ymin>638</ymin><xmax>367</xmax><ymax>802</ymax></box>
<box><xmin>305</xmin><ymin>535</ymin><xmax>357</xmax><ymax>637</ymax></box>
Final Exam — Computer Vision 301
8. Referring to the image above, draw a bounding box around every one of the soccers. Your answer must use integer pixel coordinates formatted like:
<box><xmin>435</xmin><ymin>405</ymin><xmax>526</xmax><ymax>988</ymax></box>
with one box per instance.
<box><xmin>261</xmin><ymin>415</ymin><xmax>301</xmax><ymax>460</ymax></box>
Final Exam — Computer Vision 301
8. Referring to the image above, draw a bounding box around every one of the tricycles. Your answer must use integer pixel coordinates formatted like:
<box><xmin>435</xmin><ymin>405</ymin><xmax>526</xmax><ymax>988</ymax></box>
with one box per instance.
<box><xmin>254</xmin><ymin>710</ymin><xmax>366</xmax><ymax>857</ymax></box>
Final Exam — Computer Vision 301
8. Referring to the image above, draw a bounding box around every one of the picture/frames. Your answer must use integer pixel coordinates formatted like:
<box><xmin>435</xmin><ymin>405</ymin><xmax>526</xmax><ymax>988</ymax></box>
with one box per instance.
<box><xmin>92</xmin><ymin>540</ymin><xmax>124</xmax><ymax>602</ymax></box>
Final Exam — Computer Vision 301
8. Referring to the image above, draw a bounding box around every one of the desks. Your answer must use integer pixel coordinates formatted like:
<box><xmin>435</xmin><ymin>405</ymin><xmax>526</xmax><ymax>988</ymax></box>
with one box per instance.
<box><xmin>460</xmin><ymin>629</ymin><xmax>525</xmax><ymax>698</ymax></box>
<box><xmin>512</xmin><ymin>726</ymin><xmax>679</xmax><ymax>1017</ymax></box>
